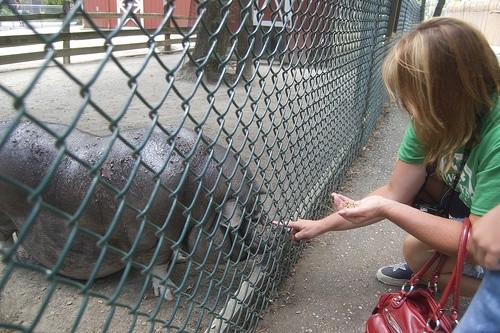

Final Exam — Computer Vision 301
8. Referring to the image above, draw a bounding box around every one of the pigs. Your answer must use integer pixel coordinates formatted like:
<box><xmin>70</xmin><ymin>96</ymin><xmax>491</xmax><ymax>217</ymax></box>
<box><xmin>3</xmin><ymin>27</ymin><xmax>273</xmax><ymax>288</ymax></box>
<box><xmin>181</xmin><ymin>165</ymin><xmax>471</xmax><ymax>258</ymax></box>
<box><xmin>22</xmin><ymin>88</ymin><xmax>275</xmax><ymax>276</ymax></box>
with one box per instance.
<box><xmin>0</xmin><ymin>121</ymin><xmax>289</xmax><ymax>301</ymax></box>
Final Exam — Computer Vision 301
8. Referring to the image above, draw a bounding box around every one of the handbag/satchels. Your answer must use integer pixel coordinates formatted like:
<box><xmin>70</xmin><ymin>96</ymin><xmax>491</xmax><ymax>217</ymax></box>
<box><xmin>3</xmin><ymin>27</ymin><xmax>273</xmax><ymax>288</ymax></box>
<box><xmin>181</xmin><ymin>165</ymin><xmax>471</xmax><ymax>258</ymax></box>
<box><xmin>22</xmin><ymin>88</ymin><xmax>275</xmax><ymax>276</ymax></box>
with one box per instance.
<box><xmin>364</xmin><ymin>218</ymin><xmax>471</xmax><ymax>333</ymax></box>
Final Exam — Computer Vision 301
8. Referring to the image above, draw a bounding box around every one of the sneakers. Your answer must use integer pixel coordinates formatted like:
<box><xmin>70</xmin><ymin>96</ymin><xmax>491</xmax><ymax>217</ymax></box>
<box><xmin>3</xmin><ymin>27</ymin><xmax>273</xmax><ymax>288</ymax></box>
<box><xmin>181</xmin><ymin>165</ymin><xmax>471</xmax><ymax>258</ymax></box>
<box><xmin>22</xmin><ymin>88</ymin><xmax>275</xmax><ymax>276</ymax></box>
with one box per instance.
<box><xmin>376</xmin><ymin>263</ymin><xmax>428</xmax><ymax>287</ymax></box>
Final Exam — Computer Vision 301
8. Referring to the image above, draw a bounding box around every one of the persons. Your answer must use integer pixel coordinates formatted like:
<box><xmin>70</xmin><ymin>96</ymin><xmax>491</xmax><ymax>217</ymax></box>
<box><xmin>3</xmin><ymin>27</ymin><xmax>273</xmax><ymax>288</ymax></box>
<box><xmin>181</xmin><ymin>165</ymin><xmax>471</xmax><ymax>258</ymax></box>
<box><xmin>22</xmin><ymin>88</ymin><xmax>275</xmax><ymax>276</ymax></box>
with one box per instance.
<box><xmin>271</xmin><ymin>16</ymin><xmax>500</xmax><ymax>333</ymax></box>
